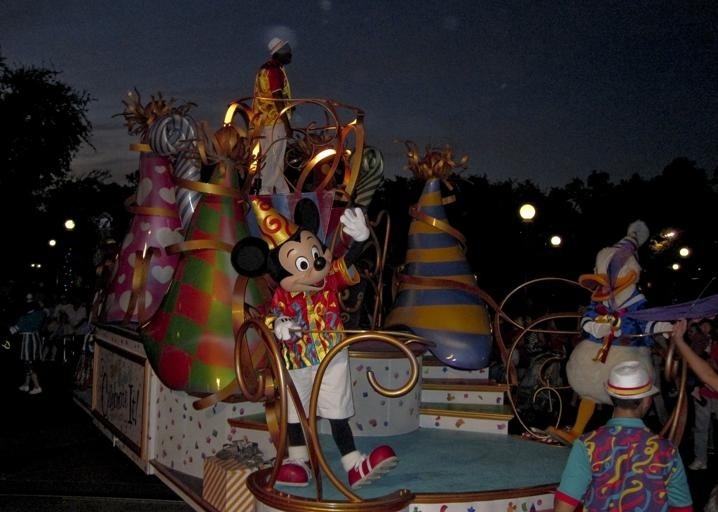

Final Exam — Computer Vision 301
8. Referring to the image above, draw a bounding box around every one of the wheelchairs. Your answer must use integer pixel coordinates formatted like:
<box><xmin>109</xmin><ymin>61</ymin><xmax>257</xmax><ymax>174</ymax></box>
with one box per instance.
<box><xmin>42</xmin><ymin>336</ymin><xmax>75</xmax><ymax>369</ymax></box>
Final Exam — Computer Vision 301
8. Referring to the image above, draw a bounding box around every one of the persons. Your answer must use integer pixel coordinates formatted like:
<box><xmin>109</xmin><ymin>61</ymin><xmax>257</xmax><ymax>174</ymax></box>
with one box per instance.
<box><xmin>10</xmin><ymin>293</ymin><xmax>89</xmax><ymax>396</ymax></box>
<box><xmin>658</xmin><ymin>317</ymin><xmax>718</xmax><ymax>470</ymax></box>
<box><xmin>554</xmin><ymin>359</ymin><xmax>694</xmax><ymax>512</ymax></box>
